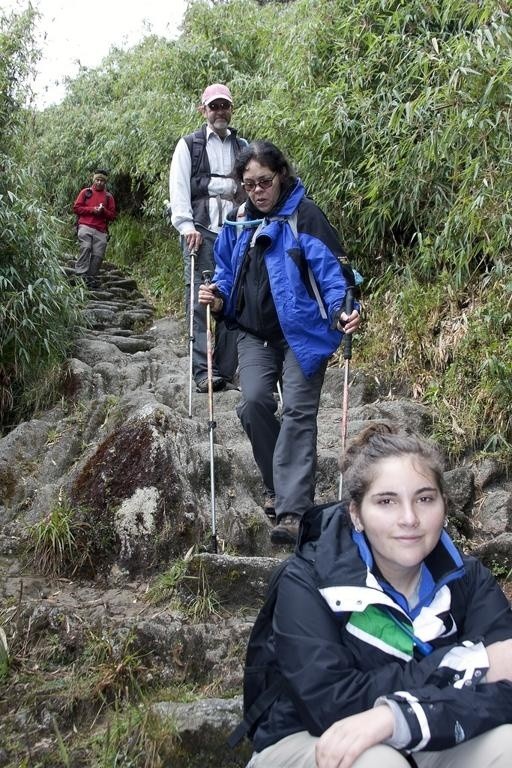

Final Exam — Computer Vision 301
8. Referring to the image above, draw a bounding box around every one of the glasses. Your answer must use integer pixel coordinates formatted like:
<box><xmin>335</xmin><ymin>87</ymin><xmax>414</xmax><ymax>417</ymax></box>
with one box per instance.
<box><xmin>241</xmin><ymin>171</ymin><xmax>277</xmax><ymax>192</ymax></box>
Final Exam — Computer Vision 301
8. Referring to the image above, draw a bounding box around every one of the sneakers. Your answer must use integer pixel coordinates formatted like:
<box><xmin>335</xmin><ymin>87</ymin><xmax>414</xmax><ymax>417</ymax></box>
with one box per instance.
<box><xmin>263</xmin><ymin>497</ymin><xmax>280</xmax><ymax>517</ymax></box>
<box><xmin>196</xmin><ymin>376</ymin><xmax>226</xmax><ymax>393</ymax></box>
<box><xmin>88</xmin><ymin>277</ymin><xmax>102</xmax><ymax>287</ymax></box>
<box><xmin>270</xmin><ymin>513</ymin><xmax>301</xmax><ymax>545</ymax></box>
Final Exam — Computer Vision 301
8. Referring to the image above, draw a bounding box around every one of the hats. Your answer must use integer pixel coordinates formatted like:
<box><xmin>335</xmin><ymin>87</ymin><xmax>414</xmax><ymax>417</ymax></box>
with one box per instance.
<box><xmin>201</xmin><ymin>83</ymin><xmax>232</xmax><ymax>106</ymax></box>
<box><xmin>93</xmin><ymin>169</ymin><xmax>109</xmax><ymax>184</ymax></box>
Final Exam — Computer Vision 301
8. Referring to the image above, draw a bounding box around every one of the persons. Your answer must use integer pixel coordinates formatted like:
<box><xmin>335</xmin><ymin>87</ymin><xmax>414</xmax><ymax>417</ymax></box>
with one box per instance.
<box><xmin>239</xmin><ymin>418</ymin><xmax>512</xmax><ymax>767</ymax></box>
<box><xmin>167</xmin><ymin>81</ymin><xmax>254</xmax><ymax>392</ymax></box>
<box><xmin>71</xmin><ymin>168</ymin><xmax>118</xmax><ymax>292</ymax></box>
<box><xmin>194</xmin><ymin>136</ymin><xmax>369</xmax><ymax>547</ymax></box>
